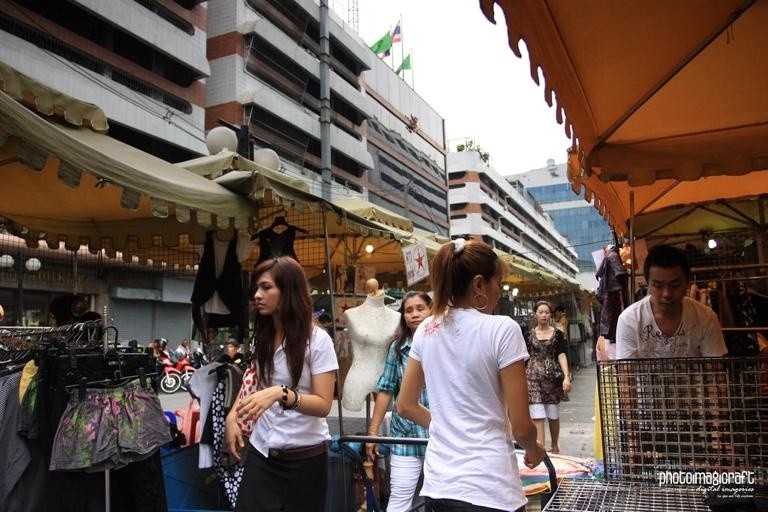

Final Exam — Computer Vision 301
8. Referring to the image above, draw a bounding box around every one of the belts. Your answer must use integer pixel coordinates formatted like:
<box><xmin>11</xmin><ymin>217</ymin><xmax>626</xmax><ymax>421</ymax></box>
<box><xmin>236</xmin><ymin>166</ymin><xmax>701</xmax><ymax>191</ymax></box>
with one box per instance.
<box><xmin>268</xmin><ymin>441</ymin><xmax>332</xmax><ymax>461</ymax></box>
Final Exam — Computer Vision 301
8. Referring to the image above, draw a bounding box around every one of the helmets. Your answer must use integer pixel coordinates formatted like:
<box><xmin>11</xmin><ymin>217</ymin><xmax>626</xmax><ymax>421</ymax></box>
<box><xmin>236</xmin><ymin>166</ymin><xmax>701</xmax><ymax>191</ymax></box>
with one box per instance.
<box><xmin>157</xmin><ymin>338</ymin><xmax>167</xmax><ymax>350</ymax></box>
<box><xmin>193</xmin><ymin>350</ymin><xmax>202</xmax><ymax>359</ymax></box>
<box><xmin>175</xmin><ymin>348</ymin><xmax>185</xmax><ymax>359</ymax></box>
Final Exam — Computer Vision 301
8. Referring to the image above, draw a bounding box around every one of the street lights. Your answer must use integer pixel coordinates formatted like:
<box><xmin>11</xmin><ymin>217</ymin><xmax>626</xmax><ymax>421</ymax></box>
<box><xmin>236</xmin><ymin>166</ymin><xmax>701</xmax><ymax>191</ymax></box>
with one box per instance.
<box><xmin>0</xmin><ymin>249</ymin><xmax>43</xmax><ymax>327</ymax></box>
<box><xmin>206</xmin><ymin>117</ymin><xmax>281</xmax><ymax>367</ymax></box>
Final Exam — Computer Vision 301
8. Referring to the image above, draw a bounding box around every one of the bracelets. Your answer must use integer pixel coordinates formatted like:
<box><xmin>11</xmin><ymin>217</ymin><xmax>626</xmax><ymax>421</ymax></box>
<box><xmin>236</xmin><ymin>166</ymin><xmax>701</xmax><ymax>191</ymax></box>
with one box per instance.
<box><xmin>368</xmin><ymin>431</ymin><xmax>381</xmax><ymax>436</ymax></box>
<box><xmin>281</xmin><ymin>385</ymin><xmax>300</xmax><ymax>409</ymax></box>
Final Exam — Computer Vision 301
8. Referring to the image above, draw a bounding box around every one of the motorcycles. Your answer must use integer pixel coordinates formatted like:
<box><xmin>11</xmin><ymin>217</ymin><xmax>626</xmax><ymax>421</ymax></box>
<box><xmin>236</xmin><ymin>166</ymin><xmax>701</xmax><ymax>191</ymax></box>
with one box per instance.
<box><xmin>154</xmin><ymin>348</ymin><xmax>210</xmax><ymax>394</ymax></box>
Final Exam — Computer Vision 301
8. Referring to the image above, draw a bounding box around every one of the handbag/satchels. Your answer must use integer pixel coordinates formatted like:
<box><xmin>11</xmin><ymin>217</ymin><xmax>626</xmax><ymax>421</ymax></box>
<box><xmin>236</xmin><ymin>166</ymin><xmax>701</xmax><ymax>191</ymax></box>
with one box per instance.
<box><xmin>235</xmin><ymin>361</ymin><xmax>260</xmax><ymax>438</ymax></box>
<box><xmin>544</xmin><ymin>359</ymin><xmax>572</xmax><ymax>384</ymax></box>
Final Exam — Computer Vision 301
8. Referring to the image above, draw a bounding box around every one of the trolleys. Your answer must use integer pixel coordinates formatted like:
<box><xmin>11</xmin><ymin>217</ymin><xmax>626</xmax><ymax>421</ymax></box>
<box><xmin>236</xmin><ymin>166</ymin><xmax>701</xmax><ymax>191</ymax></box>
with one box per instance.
<box><xmin>335</xmin><ymin>434</ymin><xmax>600</xmax><ymax>511</ymax></box>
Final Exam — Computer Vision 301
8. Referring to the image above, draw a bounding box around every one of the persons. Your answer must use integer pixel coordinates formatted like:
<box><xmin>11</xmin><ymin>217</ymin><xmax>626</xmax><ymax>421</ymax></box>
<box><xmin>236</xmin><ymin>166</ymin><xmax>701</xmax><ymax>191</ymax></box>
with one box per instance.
<box><xmin>223</xmin><ymin>256</ymin><xmax>340</xmax><ymax>512</ymax></box>
<box><xmin>148</xmin><ymin>338</ymin><xmax>192</xmax><ymax>379</ymax></box>
<box><xmin>554</xmin><ymin>306</ymin><xmax>568</xmax><ymax>334</ymax></box>
<box><xmin>342</xmin><ymin>290</ymin><xmax>402</xmax><ymax>411</ymax></box>
<box><xmin>216</xmin><ymin>339</ymin><xmax>244</xmax><ymax>369</ymax></box>
<box><xmin>395</xmin><ymin>239</ymin><xmax>545</xmax><ymax>512</ymax></box>
<box><xmin>318</xmin><ymin>313</ymin><xmax>335</xmax><ymax>341</ymax></box>
<box><xmin>615</xmin><ymin>245</ymin><xmax>745</xmax><ymax>476</ymax></box>
<box><xmin>525</xmin><ymin>300</ymin><xmax>571</xmax><ymax>454</ymax></box>
<box><xmin>365</xmin><ymin>290</ymin><xmax>434</xmax><ymax>512</ymax></box>
